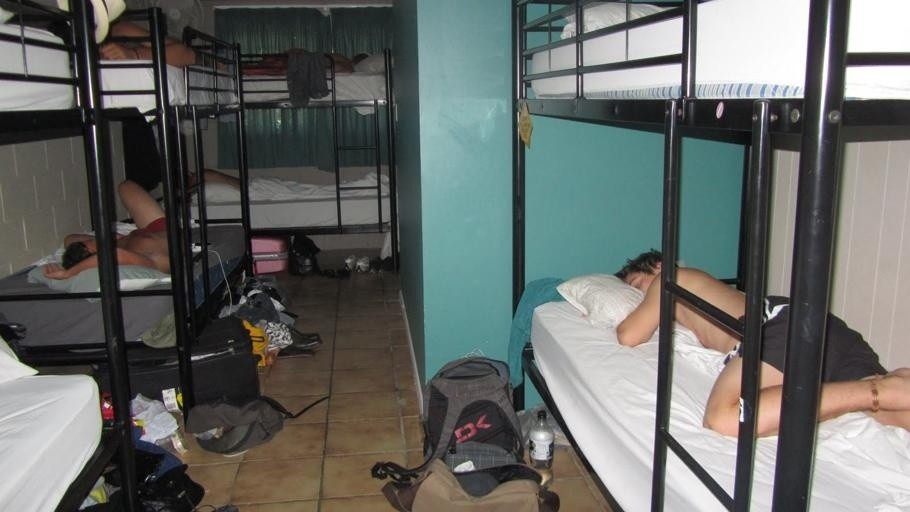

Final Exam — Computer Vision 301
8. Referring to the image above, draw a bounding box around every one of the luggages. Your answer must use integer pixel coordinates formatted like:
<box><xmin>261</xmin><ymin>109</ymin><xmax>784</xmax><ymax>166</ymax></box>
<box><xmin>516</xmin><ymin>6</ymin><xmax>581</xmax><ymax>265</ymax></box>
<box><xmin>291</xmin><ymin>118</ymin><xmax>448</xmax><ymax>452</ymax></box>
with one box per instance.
<box><xmin>92</xmin><ymin>316</ymin><xmax>260</xmax><ymax>406</ymax></box>
<box><xmin>251</xmin><ymin>238</ymin><xmax>289</xmax><ymax>275</ymax></box>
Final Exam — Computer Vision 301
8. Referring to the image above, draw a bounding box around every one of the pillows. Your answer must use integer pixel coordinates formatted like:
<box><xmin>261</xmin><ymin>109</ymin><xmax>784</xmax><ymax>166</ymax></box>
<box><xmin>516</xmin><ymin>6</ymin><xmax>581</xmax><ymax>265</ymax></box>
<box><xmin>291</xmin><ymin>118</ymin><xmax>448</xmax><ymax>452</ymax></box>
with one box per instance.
<box><xmin>557</xmin><ymin>274</ymin><xmax>646</xmax><ymax>330</ymax></box>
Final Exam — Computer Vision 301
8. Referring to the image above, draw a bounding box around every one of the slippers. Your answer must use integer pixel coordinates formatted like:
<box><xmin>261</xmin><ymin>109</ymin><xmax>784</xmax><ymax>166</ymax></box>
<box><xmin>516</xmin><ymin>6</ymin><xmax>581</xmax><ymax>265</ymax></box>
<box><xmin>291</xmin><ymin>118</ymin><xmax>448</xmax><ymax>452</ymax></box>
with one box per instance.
<box><xmin>337</xmin><ymin>268</ymin><xmax>349</xmax><ymax>283</ymax></box>
<box><xmin>320</xmin><ymin>268</ymin><xmax>335</xmax><ymax>283</ymax></box>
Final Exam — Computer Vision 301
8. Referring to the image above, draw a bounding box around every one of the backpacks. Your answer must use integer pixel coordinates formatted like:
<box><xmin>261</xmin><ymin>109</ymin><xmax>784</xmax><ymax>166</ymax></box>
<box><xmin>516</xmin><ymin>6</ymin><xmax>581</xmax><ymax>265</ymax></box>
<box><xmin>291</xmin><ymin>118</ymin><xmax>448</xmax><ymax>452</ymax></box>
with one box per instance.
<box><xmin>383</xmin><ymin>460</ymin><xmax>561</xmax><ymax>512</ymax></box>
<box><xmin>372</xmin><ymin>357</ymin><xmax>527</xmax><ymax>481</ymax></box>
<box><xmin>187</xmin><ymin>398</ymin><xmax>330</xmax><ymax>457</ymax></box>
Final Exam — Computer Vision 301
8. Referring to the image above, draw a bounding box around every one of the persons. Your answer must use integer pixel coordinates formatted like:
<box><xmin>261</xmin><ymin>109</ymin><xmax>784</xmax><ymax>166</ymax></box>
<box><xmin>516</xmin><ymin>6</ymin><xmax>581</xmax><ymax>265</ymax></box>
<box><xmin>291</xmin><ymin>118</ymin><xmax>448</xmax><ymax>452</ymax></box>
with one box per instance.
<box><xmin>241</xmin><ymin>50</ymin><xmax>370</xmax><ymax>77</ymax></box>
<box><xmin>611</xmin><ymin>248</ymin><xmax>910</xmax><ymax>443</ymax></box>
<box><xmin>91</xmin><ymin>15</ymin><xmax>225</xmax><ymax>74</ymax></box>
<box><xmin>185</xmin><ymin>168</ymin><xmax>241</xmax><ymax>195</ymax></box>
<box><xmin>41</xmin><ymin>178</ymin><xmax>185</xmax><ymax>281</ymax></box>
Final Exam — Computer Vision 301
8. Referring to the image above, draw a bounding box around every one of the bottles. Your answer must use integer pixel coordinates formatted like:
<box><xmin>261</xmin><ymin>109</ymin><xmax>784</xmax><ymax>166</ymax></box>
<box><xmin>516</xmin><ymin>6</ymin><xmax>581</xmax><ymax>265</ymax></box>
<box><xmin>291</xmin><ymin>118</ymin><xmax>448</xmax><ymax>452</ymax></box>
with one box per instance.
<box><xmin>528</xmin><ymin>409</ymin><xmax>554</xmax><ymax>475</ymax></box>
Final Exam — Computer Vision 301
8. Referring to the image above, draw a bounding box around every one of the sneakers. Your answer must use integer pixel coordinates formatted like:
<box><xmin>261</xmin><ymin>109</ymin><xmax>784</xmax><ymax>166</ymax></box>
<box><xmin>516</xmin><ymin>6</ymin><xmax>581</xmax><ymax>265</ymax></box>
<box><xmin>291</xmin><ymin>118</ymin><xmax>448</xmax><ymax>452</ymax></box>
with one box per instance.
<box><xmin>345</xmin><ymin>254</ymin><xmax>396</xmax><ymax>275</ymax></box>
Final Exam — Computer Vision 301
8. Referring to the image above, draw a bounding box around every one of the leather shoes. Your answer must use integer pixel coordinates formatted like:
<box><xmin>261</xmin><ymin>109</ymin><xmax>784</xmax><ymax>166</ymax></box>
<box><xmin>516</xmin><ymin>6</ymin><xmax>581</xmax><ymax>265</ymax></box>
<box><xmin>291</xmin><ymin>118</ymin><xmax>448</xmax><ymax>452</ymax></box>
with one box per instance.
<box><xmin>278</xmin><ymin>328</ymin><xmax>320</xmax><ymax>359</ymax></box>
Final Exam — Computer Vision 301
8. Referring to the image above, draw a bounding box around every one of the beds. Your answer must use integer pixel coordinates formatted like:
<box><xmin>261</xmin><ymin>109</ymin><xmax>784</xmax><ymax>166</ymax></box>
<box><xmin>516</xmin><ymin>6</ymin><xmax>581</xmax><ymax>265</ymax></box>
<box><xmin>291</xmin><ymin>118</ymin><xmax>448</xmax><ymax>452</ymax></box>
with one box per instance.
<box><xmin>507</xmin><ymin>0</ymin><xmax>909</xmax><ymax>510</ymax></box>
<box><xmin>2</xmin><ymin>322</ymin><xmax>142</xmax><ymax>510</ymax></box>
<box><xmin>1</xmin><ymin>4</ymin><xmax>395</xmax><ymax>147</ymax></box>
<box><xmin>2</xmin><ymin>215</ymin><xmax>266</xmax><ymax>429</ymax></box>
<box><xmin>158</xmin><ymin>165</ymin><xmax>398</xmax><ymax>265</ymax></box>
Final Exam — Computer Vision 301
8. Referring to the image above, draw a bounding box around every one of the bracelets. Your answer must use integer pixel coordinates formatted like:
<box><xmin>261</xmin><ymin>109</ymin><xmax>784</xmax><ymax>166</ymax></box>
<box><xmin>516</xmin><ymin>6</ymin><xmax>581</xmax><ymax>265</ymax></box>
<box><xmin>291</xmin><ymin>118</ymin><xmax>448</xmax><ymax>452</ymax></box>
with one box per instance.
<box><xmin>133</xmin><ymin>49</ymin><xmax>139</xmax><ymax>60</ymax></box>
